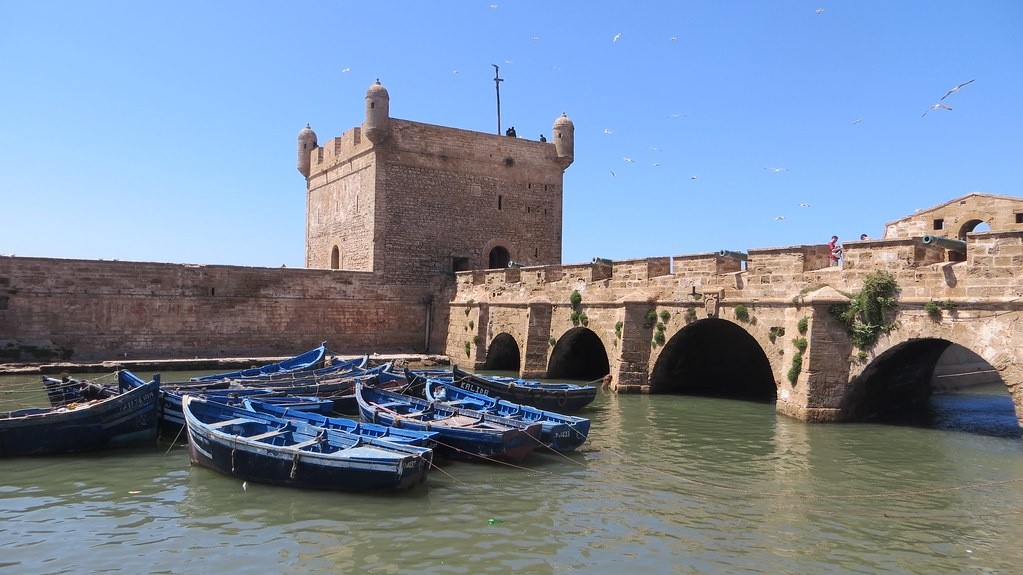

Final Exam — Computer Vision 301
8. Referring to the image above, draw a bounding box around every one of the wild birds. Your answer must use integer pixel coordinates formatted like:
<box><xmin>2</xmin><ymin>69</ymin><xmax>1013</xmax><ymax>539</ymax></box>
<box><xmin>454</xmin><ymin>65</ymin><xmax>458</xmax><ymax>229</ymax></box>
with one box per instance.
<box><xmin>799</xmin><ymin>203</ymin><xmax>810</xmax><ymax>207</ymax></box>
<box><xmin>454</xmin><ymin>71</ymin><xmax>460</xmax><ymax>74</ymax></box>
<box><xmin>610</xmin><ymin>171</ymin><xmax>616</xmax><ymax>177</ymax></box>
<box><xmin>775</xmin><ymin>217</ymin><xmax>785</xmax><ymax>220</ymax></box>
<box><xmin>666</xmin><ymin>114</ymin><xmax>687</xmax><ymax>119</ymax></box>
<box><xmin>691</xmin><ymin>177</ymin><xmax>696</xmax><ymax>180</ymax></box>
<box><xmin>532</xmin><ymin>36</ymin><xmax>538</xmax><ymax>42</ymax></box>
<box><xmin>623</xmin><ymin>157</ymin><xmax>634</xmax><ymax>163</ymax></box>
<box><xmin>941</xmin><ymin>79</ymin><xmax>974</xmax><ymax>101</ymax></box>
<box><xmin>922</xmin><ymin>104</ymin><xmax>952</xmax><ymax>116</ymax></box>
<box><xmin>342</xmin><ymin>68</ymin><xmax>351</xmax><ymax>72</ymax></box>
<box><xmin>614</xmin><ymin>32</ymin><xmax>621</xmax><ymax>42</ymax></box>
<box><xmin>491</xmin><ymin>5</ymin><xmax>498</xmax><ymax>8</ymax></box>
<box><xmin>816</xmin><ymin>8</ymin><xmax>824</xmax><ymax>14</ymax></box>
<box><xmin>763</xmin><ymin>168</ymin><xmax>790</xmax><ymax>173</ymax></box>
<box><xmin>605</xmin><ymin>129</ymin><xmax>612</xmax><ymax>135</ymax></box>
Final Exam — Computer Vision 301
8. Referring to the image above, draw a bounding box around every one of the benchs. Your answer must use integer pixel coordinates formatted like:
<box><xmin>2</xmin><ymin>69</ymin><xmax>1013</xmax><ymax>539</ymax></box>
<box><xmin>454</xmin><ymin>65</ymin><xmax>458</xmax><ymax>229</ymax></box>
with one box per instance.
<box><xmin>371</xmin><ymin>399</ymin><xmax>403</xmax><ymax>409</ymax></box>
<box><xmin>206</xmin><ymin>418</ymin><xmax>252</xmax><ymax>430</ymax></box>
<box><xmin>502</xmin><ymin>411</ymin><xmax>520</xmax><ymax>419</ymax></box>
<box><xmin>248</xmin><ymin>428</ymin><xmax>291</xmax><ymax>443</ymax></box>
<box><xmin>400</xmin><ymin>408</ymin><xmax>433</xmax><ymax>418</ymax></box>
<box><xmin>289</xmin><ymin>436</ymin><xmax>327</xmax><ymax>451</ymax></box>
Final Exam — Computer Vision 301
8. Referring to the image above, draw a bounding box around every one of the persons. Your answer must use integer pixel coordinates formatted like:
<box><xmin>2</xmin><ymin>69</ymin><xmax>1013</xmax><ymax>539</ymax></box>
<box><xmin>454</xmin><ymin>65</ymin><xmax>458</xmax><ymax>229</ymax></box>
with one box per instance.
<box><xmin>829</xmin><ymin>235</ymin><xmax>841</xmax><ymax>267</ymax></box>
<box><xmin>506</xmin><ymin>127</ymin><xmax>517</xmax><ymax>137</ymax></box>
<box><xmin>540</xmin><ymin>134</ymin><xmax>546</xmax><ymax>142</ymax></box>
<box><xmin>860</xmin><ymin>234</ymin><xmax>871</xmax><ymax>240</ymax></box>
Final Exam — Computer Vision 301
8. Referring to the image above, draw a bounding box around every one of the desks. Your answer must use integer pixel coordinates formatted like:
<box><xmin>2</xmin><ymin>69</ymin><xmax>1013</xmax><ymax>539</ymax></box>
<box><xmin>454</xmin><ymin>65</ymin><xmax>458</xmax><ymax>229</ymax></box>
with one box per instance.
<box><xmin>473</xmin><ymin>406</ymin><xmax>495</xmax><ymax>412</ymax></box>
<box><xmin>444</xmin><ymin>398</ymin><xmax>473</xmax><ymax>405</ymax></box>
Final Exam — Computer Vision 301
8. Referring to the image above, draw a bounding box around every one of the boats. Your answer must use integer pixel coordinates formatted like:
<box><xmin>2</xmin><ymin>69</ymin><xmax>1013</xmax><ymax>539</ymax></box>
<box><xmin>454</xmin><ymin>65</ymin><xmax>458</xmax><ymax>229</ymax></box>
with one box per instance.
<box><xmin>181</xmin><ymin>394</ymin><xmax>434</xmax><ymax>492</ymax></box>
<box><xmin>453</xmin><ymin>363</ymin><xmax>597</xmax><ymax>414</ymax></box>
<box><xmin>354</xmin><ymin>382</ymin><xmax>543</xmax><ymax>463</ymax></box>
<box><xmin>424</xmin><ymin>379</ymin><xmax>591</xmax><ymax>455</ymax></box>
<box><xmin>42</xmin><ymin>341</ymin><xmax>395</xmax><ymax>441</ymax></box>
<box><xmin>0</xmin><ymin>373</ymin><xmax>160</xmax><ymax>458</ymax></box>
<box><xmin>240</xmin><ymin>396</ymin><xmax>441</xmax><ymax>465</ymax></box>
